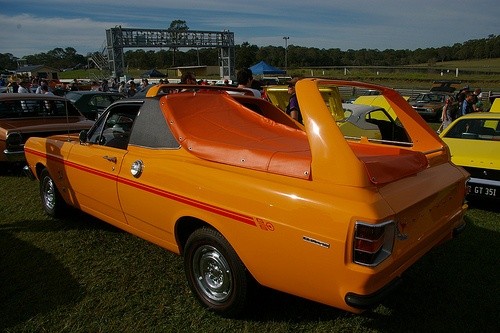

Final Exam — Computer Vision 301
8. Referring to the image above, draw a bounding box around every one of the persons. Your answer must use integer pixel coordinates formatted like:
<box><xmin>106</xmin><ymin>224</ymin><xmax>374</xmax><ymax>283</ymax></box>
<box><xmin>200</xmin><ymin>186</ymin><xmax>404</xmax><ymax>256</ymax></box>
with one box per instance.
<box><xmin>6</xmin><ymin>69</ymin><xmax>271</xmax><ymax>112</ymax></box>
<box><xmin>436</xmin><ymin>86</ymin><xmax>483</xmax><ymax>135</ymax></box>
<box><xmin>285</xmin><ymin>76</ymin><xmax>303</xmax><ymax>125</ymax></box>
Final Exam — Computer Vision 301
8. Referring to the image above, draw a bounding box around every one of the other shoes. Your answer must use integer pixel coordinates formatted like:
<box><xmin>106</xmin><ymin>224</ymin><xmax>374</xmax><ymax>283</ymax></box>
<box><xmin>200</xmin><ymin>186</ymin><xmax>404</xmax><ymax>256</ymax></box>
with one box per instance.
<box><xmin>436</xmin><ymin>130</ymin><xmax>442</xmax><ymax>134</ymax></box>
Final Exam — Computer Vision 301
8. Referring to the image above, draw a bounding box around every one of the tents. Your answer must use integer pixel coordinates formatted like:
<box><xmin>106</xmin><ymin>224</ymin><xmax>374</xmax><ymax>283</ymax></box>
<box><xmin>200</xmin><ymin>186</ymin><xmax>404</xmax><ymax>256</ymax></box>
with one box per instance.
<box><xmin>248</xmin><ymin>61</ymin><xmax>287</xmax><ymax>77</ymax></box>
<box><xmin>141</xmin><ymin>68</ymin><xmax>168</xmax><ymax>78</ymax></box>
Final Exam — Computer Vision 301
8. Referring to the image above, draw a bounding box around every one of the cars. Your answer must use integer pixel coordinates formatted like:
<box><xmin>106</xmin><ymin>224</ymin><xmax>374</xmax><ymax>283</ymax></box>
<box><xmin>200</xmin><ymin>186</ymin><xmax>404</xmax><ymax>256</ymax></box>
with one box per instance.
<box><xmin>0</xmin><ymin>87</ymin><xmax>128</xmax><ymax>163</ymax></box>
<box><xmin>22</xmin><ymin>76</ymin><xmax>474</xmax><ymax>316</ymax></box>
<box><xmin>437</xmin><ymin>97</ymin><xmax>500</xmax><ymax>203</ymax></box>
<box><xmin>406</xmin><ymin>91</ymin><xmax>452</xmax><ymax>121</ymax></box>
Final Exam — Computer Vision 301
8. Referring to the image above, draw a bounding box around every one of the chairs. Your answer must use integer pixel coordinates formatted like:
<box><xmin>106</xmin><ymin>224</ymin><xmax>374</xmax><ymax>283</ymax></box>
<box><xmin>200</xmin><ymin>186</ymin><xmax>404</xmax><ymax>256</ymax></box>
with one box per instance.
<box><xmin>478</xmin><ymin>126</ymin><xmax>496</xmax><ymax>135</ymax></box>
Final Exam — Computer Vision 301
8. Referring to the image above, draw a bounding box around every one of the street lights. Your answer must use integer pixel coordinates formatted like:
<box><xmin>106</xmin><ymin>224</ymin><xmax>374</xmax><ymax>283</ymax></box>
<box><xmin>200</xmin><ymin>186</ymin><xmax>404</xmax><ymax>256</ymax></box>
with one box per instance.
<box><xmin>282</xmin><ymin>36</ymin><xmax>290</xmax><ymax>68</ymax></box>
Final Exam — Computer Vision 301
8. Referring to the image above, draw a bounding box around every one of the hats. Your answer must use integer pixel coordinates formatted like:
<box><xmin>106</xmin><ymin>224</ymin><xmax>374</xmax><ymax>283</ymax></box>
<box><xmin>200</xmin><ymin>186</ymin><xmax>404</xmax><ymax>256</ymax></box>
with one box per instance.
<box><xmin>18</xmin><ymin>78</ymin><xmax>30</xmax><ymax>85</ymax></box>
<box><xmin>466</xmin><ymin>92</ymin><xmax>475</xmax><ymax>96</ymax></box>
<box><xmin>287</xmin><ymin>77</ymin><xmax>301</xmax><ymax>83</ymax></box>
